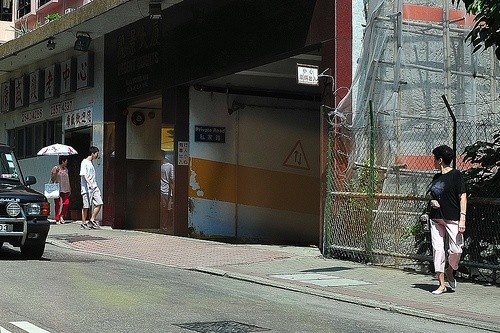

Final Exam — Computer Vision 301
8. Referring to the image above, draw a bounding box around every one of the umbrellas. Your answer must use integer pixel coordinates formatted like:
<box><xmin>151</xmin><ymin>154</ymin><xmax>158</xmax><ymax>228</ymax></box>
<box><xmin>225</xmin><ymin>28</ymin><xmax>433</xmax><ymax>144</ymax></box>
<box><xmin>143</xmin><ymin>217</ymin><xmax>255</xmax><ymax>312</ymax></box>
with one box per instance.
<box><xmin>37</xmin><ymin>145</ymin><xmax>78</xmax><ymax>167</ymax></box>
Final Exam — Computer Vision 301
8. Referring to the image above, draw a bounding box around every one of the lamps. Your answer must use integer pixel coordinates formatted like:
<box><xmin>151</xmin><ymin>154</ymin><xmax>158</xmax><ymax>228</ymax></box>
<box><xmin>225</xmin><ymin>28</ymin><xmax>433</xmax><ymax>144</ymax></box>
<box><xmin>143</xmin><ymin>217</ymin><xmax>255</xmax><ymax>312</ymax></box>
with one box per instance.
<box><xmin>74</xmin><ymin>35</ymin><xmax>92</xmax><ymax>52</ymax></box>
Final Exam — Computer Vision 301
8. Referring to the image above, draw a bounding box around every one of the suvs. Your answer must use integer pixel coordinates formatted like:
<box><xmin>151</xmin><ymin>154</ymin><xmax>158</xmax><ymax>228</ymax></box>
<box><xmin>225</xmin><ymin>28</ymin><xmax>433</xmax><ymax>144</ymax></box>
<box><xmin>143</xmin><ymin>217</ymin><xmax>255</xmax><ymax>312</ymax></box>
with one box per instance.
<box><xmin>1</xmin><ymin>144</ymin><xmax>51</xmax><ymax>258</ymax></box>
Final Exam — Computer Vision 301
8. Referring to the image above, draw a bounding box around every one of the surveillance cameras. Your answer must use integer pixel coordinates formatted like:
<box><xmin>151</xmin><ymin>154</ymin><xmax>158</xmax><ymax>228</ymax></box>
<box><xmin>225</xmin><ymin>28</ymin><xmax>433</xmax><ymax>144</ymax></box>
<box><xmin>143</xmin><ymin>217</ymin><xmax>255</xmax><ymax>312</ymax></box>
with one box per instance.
<box><xmin>232</xmin><ymin>100</ymin><xmax>245</xmax><ymax>110</ymax></box>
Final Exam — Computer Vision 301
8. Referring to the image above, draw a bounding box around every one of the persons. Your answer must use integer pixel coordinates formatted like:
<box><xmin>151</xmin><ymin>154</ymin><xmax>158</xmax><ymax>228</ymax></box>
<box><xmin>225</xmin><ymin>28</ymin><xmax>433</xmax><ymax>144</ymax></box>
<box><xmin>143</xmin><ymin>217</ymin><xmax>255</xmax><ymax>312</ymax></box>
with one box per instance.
<box><xmin>79</xmin><ymin>146</ymin><xmax>104</xmax><ymax>232</ymax></box>
<box><xmin>159</xmin><ymin>149</ymin><xmax>175</xmax><ymax>231</ymax></box>
<box><xmin>421</xmin><ymin>145</ymin><xmax>469</xmax><ymax>296</ymax></box>
<box><xmin>48</xmin><ymin>156</ymin><xmax>72</xmax><ymax>224</ymax></box>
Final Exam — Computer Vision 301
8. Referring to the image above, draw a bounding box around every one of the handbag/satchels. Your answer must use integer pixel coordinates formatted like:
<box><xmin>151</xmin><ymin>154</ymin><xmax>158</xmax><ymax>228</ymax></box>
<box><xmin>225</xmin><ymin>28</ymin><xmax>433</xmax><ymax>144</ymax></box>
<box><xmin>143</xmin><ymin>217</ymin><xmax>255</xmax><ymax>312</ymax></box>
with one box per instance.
<box><xmin>44</xmin><ymin>177</ymin><xmax>60</xmax><ymax>199</ymax></box>
<box><xmin>420</xmin><ymin>210</ymin><xmax>431</xmax><ymax>232</ymax></box>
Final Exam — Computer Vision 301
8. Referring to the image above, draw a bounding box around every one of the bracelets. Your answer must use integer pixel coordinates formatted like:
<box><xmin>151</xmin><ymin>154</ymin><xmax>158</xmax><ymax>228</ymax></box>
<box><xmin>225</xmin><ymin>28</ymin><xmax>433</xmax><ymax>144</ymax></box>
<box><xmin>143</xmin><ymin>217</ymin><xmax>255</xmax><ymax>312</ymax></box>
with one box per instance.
<box><xmin>459</xmin><ymin>211</ymin><xmax>464</xmax><ymax>215</ymax></box>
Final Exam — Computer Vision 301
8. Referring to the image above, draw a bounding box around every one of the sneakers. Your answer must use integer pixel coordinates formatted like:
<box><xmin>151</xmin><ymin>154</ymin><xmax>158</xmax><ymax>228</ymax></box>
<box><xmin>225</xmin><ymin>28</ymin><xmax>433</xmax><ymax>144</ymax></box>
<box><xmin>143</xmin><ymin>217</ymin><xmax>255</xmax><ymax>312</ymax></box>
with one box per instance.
<box><xmin>88</xmin><ymin>220</ymin><xmax>100</xmax><ymax>229</ymax></box>
<box><xmin>80</xmin><ymin>223</ymin><xmax>92</xmax><ymax>229</ymax></box>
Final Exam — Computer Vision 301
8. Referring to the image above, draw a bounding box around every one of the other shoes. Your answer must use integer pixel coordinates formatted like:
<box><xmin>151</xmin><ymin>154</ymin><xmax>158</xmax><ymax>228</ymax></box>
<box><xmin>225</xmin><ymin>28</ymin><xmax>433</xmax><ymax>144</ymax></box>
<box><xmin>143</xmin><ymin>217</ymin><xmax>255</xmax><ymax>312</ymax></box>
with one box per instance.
<box><xmin>56</xmin><ymin>219</ymin><xmax>60</xmax><ymax>224</ymax></box>
<box><xmin>60</xmin><ymin>215</ymin><xmax>65</xmax><ymax>224</ymax></box>
<box><xmin>432</xmin><ymin>286</ymin><xmax>449</xmax><ymax>294</ymax></box>
<box><xmin>162</xmin><ymin>225</ymin><xmax>168</xmax><ymax>232</ymax></box>
<box><xmin>445</xmin><ymin>268</ymin><xmax>457</xmax><ymax>289</ymax></box>
